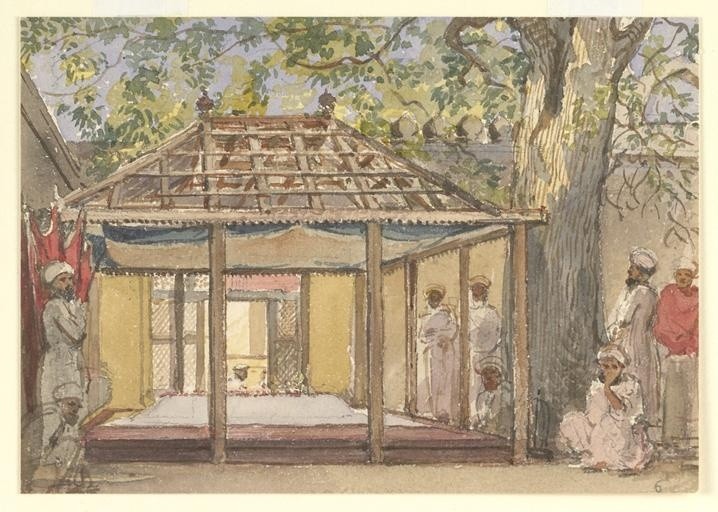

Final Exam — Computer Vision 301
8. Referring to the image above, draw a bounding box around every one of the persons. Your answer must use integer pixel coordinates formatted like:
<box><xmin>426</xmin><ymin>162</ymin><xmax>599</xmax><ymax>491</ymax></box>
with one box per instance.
<box><xmin>652</xmin><ymin>259</ymin><xmax>700</xmax><ymax>446</ymax></box>
<box><xmin>20</xmin><ymin>382</ymin><xmax>94</xmax><ymax>494</ymax></box>
<box><xmin>35</xmin><ymin>258</ymin><xmax>85</xmax><ymax>427</ymax></box>
<box><xmin>467</xmin><ymin>272</ymin><xmax>505</xmax><ymax>411</ymax></box>
<box><xmin>607</xmin><ymin>245</ymin><xmax>661</xmax><ymax>431</ymax></box>
<box><xmin>474</xmin><ymin>356</ymin><xmax>511</xmax><ymax>430</ymax></box>
<box><xmin>228</xmin><ymin>363</ymin><xmax>249</xmax><ymax>389</ymax></box>
<box><xmin>559</xmin><ymin>345</ymin><xmax>649</xmax><ymax>478</ymax></box>
<box><xmin>417</xmin><ymin>283</ymin><xmax>456</xmax><ymax>419</ymax></box>
